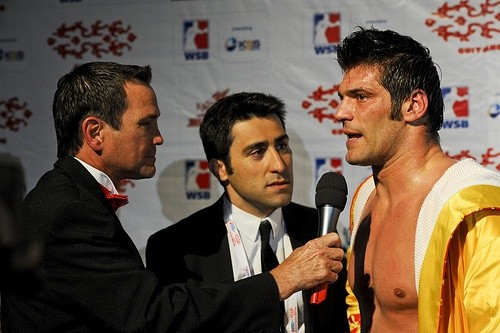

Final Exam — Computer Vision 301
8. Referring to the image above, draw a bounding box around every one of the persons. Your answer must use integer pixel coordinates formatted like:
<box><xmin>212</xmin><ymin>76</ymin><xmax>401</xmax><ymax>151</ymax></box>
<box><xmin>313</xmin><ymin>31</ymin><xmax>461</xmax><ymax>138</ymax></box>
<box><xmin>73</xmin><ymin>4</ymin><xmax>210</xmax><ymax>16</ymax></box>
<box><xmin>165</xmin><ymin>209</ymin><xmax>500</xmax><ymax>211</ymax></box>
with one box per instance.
<box><xmin>145</xmin><ymin>91</ymin><xmax>349</xmax><ymax>333</ymax></box>
<box><xmin>334</xmin><ymin>29</ymin><xmax>500</xmax><ymax>333</ymax></box>
<box><xmin>23</xmin><ymin>61</ymin><xmax>343</xmax><ymax>333</ymax></box>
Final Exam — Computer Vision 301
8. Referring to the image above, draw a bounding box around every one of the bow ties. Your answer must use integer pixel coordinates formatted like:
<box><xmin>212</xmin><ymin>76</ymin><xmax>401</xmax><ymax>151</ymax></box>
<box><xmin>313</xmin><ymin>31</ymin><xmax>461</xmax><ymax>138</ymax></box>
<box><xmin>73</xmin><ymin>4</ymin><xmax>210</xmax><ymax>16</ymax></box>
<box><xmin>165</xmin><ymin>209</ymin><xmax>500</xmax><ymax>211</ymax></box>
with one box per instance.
<box><xmin>100</xmin><ymin>183</ymin><xmax>130</xmax><ymax>214</ymax></box>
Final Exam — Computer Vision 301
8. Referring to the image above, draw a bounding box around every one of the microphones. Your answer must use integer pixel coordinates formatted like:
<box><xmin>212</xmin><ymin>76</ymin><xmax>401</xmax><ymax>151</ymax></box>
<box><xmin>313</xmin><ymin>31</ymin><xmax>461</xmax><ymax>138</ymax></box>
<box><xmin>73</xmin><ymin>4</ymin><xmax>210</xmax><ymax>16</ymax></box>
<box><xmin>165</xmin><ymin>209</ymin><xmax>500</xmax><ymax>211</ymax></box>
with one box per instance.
<box><xmin>310</xmin><ymin>172</ymin><xmax>348</xmax><ymax>305</ymax></box>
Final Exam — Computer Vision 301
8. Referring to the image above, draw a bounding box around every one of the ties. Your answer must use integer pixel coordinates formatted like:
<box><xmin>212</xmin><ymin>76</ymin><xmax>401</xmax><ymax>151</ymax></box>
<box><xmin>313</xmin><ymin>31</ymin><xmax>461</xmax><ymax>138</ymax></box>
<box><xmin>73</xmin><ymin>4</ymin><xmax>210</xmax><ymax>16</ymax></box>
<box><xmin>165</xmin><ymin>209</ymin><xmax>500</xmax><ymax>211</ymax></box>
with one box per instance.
<box><xmin>259</xmin><ymin>220</ymin><xmax>285</xmax><ymax>333</ymax></box>
